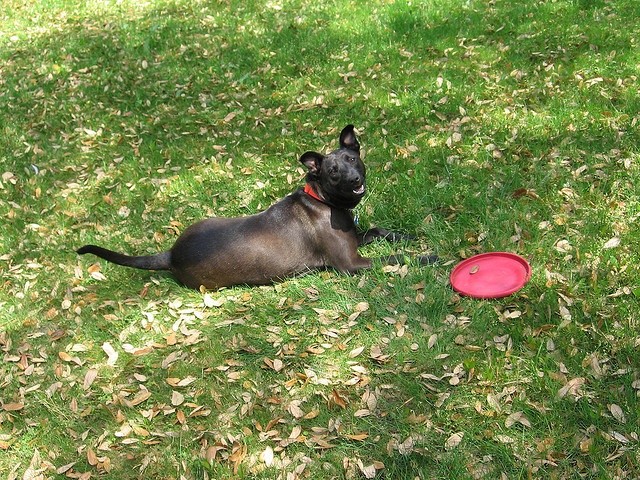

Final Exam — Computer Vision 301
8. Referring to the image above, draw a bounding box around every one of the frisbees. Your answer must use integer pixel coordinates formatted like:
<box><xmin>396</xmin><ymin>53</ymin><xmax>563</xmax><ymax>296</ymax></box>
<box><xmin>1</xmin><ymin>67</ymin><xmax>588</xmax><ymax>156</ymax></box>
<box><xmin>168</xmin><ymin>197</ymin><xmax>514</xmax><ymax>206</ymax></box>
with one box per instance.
<box><xmin>450</xmin><ymin>252</ymin><xmax>530</xmax><ymax>299</ymax></box>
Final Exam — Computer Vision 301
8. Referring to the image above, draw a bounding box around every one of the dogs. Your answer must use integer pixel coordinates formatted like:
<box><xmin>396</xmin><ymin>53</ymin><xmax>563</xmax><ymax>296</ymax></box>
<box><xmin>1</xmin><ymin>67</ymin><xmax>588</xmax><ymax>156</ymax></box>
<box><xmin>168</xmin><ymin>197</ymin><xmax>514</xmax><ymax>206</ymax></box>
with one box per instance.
<box><xmin>76</xmin><ymin>124</ymin><xmax>438</xmax><ymax>293</ymax></box>
